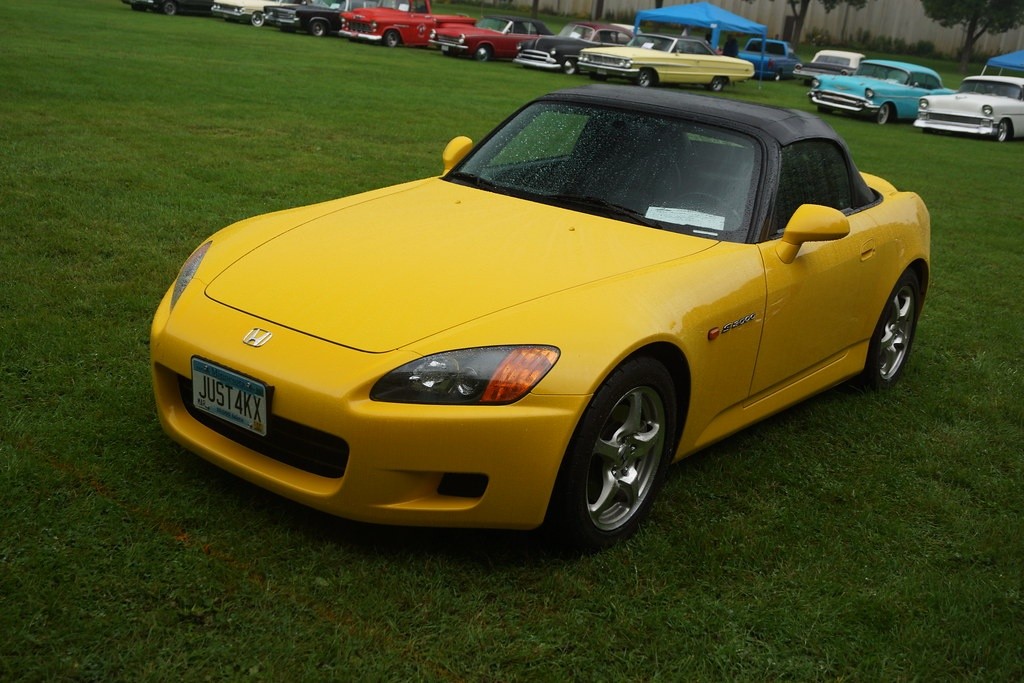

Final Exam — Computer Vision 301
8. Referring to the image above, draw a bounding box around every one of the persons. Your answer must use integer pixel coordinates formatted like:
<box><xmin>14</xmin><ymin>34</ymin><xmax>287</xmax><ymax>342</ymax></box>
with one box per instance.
<box><xmin>703</xmin><ymin>33</ymin><xmax>712</xmax><ymax>45</ymax></box>
<box><xmin>722</xmin><ymin>33</ymin><xmax>740</xmax><ymax>57</ymax></box>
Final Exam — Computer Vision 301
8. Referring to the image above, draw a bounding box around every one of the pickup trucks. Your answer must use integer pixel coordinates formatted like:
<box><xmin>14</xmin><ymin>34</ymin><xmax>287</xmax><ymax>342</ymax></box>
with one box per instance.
<box><xmin>338</xmin><ymin>0</ymin><xmax>478</xmax><ymax>48</ymax></box>
<box><xmin>739</xmin><ymin>37</ymin><xmax>800</xmax><ymax>81</ymax></box>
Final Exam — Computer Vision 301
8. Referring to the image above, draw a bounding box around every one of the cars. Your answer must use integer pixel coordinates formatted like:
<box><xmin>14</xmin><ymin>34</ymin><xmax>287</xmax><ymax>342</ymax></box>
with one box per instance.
<box><xmin>121</xmin><ymin>0</ymin><xmax>214</xmax><ymax>16</ymax></box>
<box><xmin>790</xmin><ymin>51</ymin><xmax>868</xmax><ymax>86</ymax></box>
<box><xmin>147</xmin><ymin>84</ymin><xmax>931</xmax><ymax>549</ymax></box>
<box><xmin>578</xmin><ymin>33</ymin><xmax>756</xmax><ymax>87</ymax></box>
<box><xmin>425</xmin><ymin>15</ymin><xmax>555</xmax><ymax>61</ymax></box>
<box><xmin>806</xmin><ymin>60</ymin><xmax>956</xmax><ymax>126</ymax></box>
<box><xmin>510</xmin><ymin>20</ymin><xmax>634</xmax><ymax>75</ymax></box>
<box><xmin>263</xmin><ymin>0</ymin><xmax>345</xmax><ymax>38</ymax></box>
<box><xmin>911</xmin><ymin>75</ymin><xmax>1024</xmax><ymax>146</ymax></box>
<box><xmin>209</xmin><ymin>0</ymin><xmax>307</xmax><ymax>27</ymax></box>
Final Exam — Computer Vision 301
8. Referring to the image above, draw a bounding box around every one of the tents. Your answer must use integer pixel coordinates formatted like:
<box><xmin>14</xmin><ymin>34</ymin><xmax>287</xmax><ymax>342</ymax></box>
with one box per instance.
<box><xmin>633</xmin><ymin>1</ymin><xmax>768</xmax><ymax>90</ymax></box>
<box><xmin>974</xmin><ymin>49</ymin><xmax>1024</xmax><ymax>92</ymax></box>
